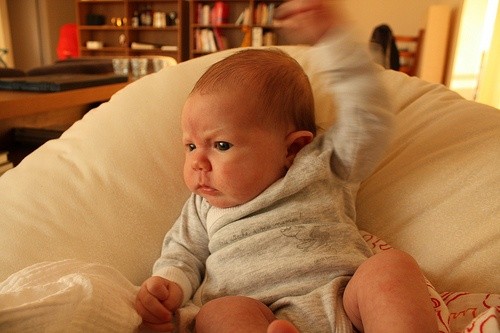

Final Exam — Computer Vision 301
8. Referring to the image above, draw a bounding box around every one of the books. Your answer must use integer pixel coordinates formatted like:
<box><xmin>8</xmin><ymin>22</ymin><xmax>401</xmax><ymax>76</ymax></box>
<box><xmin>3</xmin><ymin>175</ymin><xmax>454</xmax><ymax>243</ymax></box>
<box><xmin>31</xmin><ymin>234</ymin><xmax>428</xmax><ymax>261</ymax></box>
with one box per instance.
<box><xmin>131</xmin><ymin>2</ymin><xmax>275</xmax><ymax>51</ymax></box>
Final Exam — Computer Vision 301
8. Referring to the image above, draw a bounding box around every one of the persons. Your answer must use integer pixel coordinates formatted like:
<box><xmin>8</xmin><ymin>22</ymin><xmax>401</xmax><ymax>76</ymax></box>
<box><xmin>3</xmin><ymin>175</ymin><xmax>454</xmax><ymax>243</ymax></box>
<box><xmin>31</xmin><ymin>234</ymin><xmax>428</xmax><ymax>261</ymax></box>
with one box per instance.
<box><xmin>130</xmin><ymin>0</ymin><xmax>440</xmax><ymax>333</ymax></box>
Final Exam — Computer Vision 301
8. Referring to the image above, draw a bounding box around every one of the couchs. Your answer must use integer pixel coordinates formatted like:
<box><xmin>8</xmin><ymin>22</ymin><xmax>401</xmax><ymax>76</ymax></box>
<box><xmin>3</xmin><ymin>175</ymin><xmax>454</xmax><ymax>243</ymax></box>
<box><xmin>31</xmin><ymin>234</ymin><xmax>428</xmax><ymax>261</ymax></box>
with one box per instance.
<box><xmin>0</xmin><ymin>45</ymin><xmax>500</xmax><ymax>333</ymax></box>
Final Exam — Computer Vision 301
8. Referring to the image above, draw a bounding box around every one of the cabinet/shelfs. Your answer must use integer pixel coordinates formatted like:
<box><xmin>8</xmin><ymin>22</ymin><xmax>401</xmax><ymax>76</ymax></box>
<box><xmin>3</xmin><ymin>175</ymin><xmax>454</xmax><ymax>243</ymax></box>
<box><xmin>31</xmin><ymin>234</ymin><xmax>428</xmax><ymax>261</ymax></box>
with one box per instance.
<box><xmin>189</xmin><ymin>0</ymin><xmax>286</xmax><ymax>61</ymax></box>
<box><xmin>75</xmin><ymin>0</ymin><xmax>189</xmax><ymax>64</ymax></box>
